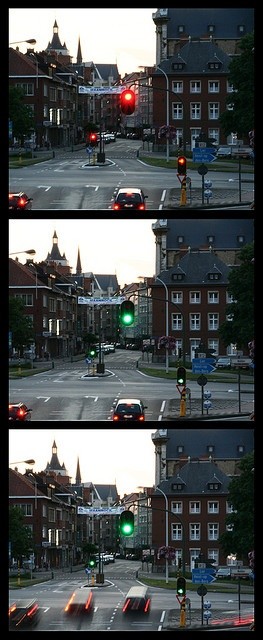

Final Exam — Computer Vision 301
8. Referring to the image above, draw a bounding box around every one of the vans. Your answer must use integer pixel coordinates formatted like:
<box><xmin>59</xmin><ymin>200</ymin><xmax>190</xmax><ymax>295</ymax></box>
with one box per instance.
<box><xmin>123</xmin><ymin>586</ymin><xmax>152</xmax><ymax>616</ymax></box>
<box><xmin>65</xmin><ymin>589</ymin><xmax>94</xmax><ymax>616</ymax></box>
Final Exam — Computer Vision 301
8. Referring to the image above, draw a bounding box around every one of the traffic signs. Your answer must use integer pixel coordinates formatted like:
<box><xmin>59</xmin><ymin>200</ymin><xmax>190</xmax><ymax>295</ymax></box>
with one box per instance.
<box><xmin>85</xmin><ymin>567</ymin><xmax>92</xmax><ymax>575</ymax></box>
<box><xmin>192</xmin><ymin>148</ymin><xmax>218</xmax><ymax>163</ymax></box>
<box><xmin>192</xmin><ymin>568</ymin><xmax>217</xmax><ymax>584</ymax></box>
<box><xmin>192</xmin><ymin>358</ymin><xmax>217</xmax><ymax>375</ymax></box>
<box><xmin>86</xmin><ymin>147</ymin><xmax>93</xmax><ymax>154</ymax></box>
<box><xmin>194</xmin><ymin>558</ymin><xmax>216</xmax><ymax>568</ymax></box>
<box><xmin>195</xmin><ymin>136</ymin><xmax>217</xmax><ymax>148</ymax></box>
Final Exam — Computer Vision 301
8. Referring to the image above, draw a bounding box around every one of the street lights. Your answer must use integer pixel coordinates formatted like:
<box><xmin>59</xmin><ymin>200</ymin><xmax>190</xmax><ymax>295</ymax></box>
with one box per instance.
<box><xmin>8</xmin><ymin>459</ymin><xmax>35</xmax><ymax>468</ymax></box>
<box><xmin>138</xmin><ymin>66</ymin><xmax>169</xmax><ymax>163</ymax></box>
<box><xmin>136</xmin><ymin>274</ymin><xmax>169</xmax><ymax>373</ymax></box>
<box><xmin>9</xmin><ymin>249</ymin><xmax>36</xmax><ymax>257</ymax></box>
<box><xmin>9</xmin><ymin>39</ymin><xmax>36</xmax><ymax>45</ymax></box>
<box><xmin>137</xmin><ymin>486</ymin><xmax>169</xmax><ymax>584</ymax></box>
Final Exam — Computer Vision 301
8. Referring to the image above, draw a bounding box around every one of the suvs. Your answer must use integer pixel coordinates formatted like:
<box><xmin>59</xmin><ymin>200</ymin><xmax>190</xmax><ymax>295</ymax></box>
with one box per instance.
<box><xmin>8</xmin><ymin>191</ymin><xmax>34</xmax><ymax>210</ymax></box>
<box><xmin>9</xmin><ymin>599</ymin><xmax>41</xmax><ymax>631</ymax></box>
<box><xmin>113</xmin><ymin>187</ymin><xmax>148</xmax><ymax>211</ymax></box>
<box><xmin>111</xmin><ymin>399</ymin><xmax>148</xmax><ymax>421</ymax></box>
<box><xmin>9</xmin><ymin>402</ymin><xmax>33</xmax><ymax>421</ymax></box>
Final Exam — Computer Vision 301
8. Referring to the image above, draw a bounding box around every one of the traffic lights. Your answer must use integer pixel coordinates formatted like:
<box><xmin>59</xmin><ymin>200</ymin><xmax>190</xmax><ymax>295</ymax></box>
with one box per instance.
<box><xmin>121</xmin><ymin>89</ymin><xmax>136</xmax><ymax>115</ymax></box>
<box><xmin>121</xmin><ymin>300</ymin><xmax>134</xmax><ymax>326</ymax></box>
<box><xmin>176</xmin><ymin>366</ymin><xmax>186</xmax><ymax>386</ymax></box>
<box><xmin>177</xmin><ymin>577</ymin><xmax>186</xmax><ymax>596</ymax></box>
<box><xmin>90</xmin><ymin>555</ymin><xmax>96</xmax><ymax>567</ymax></box>
<box><xmin>177</xmin><ymin>156</ymin><xmax>186</xmax><ymax>175</ymax></box>
<box><xmin>90</xmin><ymin>345</ymin><xmax>96</xmax><ymax>357</ymax></box>
<box><xmin>120</xmin><ymin>510</ymin><xmax>134</xmax><ymax>536</ymax></box>
<box><xmin>90</xmin><ymin>133</ymin><xmax>97</xmax><ymax>145</ymax></box>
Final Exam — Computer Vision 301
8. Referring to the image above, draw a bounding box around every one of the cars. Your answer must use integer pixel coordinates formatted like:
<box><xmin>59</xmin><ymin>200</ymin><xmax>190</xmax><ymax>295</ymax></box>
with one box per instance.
<box><xmin>102</xmin><ymin>135</ymin><xmax>111</xmax><ymax>143</ymax></box>
<box><xmin>107</xmin><ymin>345</ymin><xmax>115</xmax><ymax>352</ymax></box>
<box><xmin>215</xmin><ymin>357</ymin><xmax>232</xmax><ymax>370</ymax></box>
<box><xmin>216</xmin><ymin>145</ymin><xmax>235</xmax><ymax>159</ymax></box>
<box><xmin>216</xmin><ymin>568</ymin><xmax>232</xmax><ymax>579</ymax></box>
<box><xmin>110</xmin><ymin>135</ymin><xmax>116</xmax><ymax>142</ymax></box>
<box><xmin>105</xmin><ymin>555</ymin><xmax>114</xmax><ymax>563</ymax></box>
